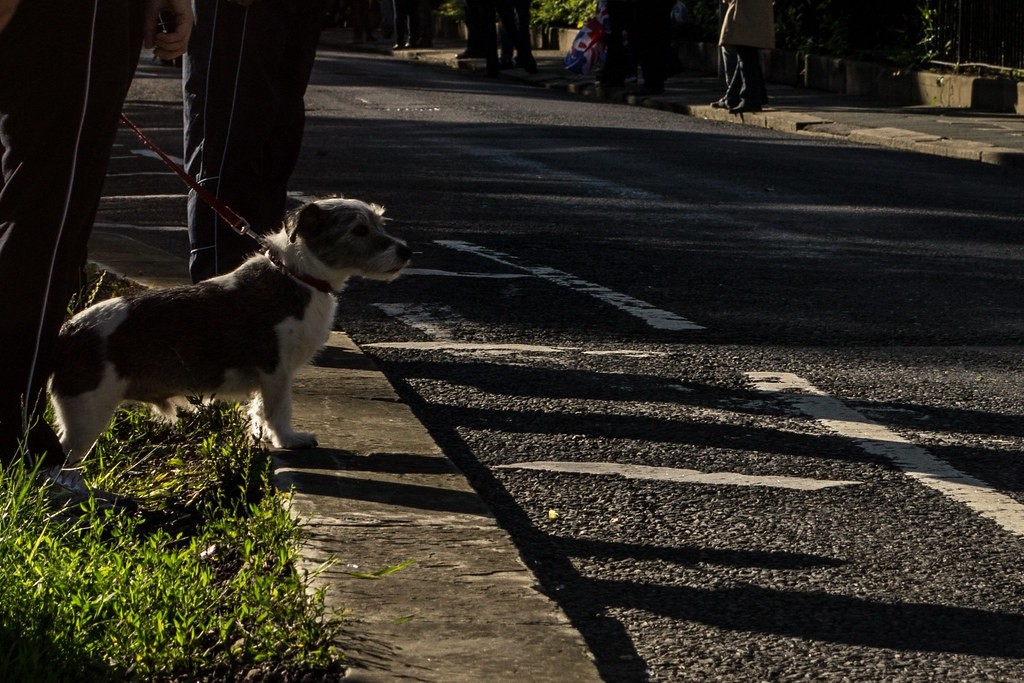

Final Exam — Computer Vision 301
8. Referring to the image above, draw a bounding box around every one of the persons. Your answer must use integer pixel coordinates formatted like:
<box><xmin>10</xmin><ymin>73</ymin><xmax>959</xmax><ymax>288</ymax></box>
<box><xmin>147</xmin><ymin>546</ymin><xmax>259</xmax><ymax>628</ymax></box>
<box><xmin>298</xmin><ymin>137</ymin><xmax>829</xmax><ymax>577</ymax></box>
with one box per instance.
<box><xmin>594</xmin><ymin>0</ymin><xmax>672</xmax><ymax>94</ymax></box>
<box><xmin>0</xmin><ymin>0</ymin><xmax>198</xmax><ymax>503</ymax></box>
<box><xmin>493</xmin><ymin>0</ymin><xmax>539</xmax><ymax>76</ymax></box>
<box><xmin>390</xmin><ymin>0</ymin><xmax>420</xmax><ymax>50</ymax></box>
<box><xmin>350</xmin><ymin>0</ymin><xmax>378</xmax><ymax>47</ymax></box>
<box><xmin>708</xmin><ymin>0</ymin><xmax>774</xmax><ymax>113</ymax></box>
<box><xmin>455</xmin><ymin>0</ymin><xmax>500</xmax><ymax>67</ymax></box>
<box><xmin>179</xmin><ymin>2</ymin><xmax>326</xmax><ymax>281</ymax></box>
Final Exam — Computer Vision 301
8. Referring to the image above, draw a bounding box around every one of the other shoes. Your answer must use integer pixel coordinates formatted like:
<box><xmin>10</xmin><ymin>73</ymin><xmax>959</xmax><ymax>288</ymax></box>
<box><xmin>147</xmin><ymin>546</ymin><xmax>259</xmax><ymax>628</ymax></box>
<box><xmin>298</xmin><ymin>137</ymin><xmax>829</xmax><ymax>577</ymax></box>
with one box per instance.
<box><xmin>711</xmin><ymin>98</ymin><xmax>759</xmax><ymax>114</ymax></box>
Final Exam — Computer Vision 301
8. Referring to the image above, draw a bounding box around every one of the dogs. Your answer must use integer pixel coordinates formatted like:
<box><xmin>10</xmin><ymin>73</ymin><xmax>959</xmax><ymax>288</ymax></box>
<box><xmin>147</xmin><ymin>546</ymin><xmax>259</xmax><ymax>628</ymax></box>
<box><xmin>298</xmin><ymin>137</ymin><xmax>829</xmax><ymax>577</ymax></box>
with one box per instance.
<box><xmin>37</xmin><ymin>197</ymin><xmax>414</xmax><ymax>469</ymax></box>
